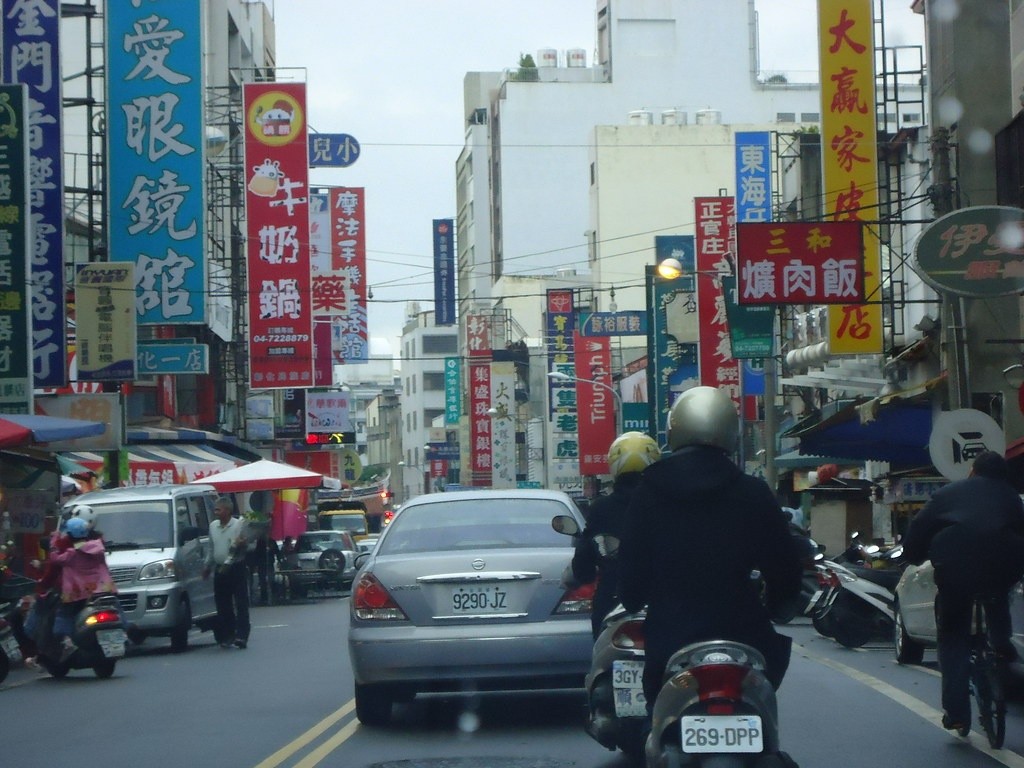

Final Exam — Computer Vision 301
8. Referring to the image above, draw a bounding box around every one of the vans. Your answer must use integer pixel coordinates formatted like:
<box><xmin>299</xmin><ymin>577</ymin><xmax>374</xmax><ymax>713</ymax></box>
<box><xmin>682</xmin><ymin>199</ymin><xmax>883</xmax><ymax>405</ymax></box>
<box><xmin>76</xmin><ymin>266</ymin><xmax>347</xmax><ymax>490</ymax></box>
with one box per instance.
<box><xmin>57</xmin><ymin>483</ymin><xmax>228</xmax><ymax>654</ymax></box>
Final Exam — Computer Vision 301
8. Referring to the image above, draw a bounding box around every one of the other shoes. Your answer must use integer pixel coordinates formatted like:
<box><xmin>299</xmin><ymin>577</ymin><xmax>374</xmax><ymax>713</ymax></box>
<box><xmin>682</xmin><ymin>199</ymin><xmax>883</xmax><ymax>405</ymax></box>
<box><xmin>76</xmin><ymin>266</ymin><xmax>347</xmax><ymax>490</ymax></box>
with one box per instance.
<box><xmin>942</xmin><ymin>712</ymin><xmax>952</xmax><ymax>727</ymax></box>
<box><xmin>234</xmin><ymin>639</ymin><xmax>246</xmax><ymax>648</ymax></box>
<box><xmin>59</xmin><ymin>645</ymin><xmax>79</xmax><ymax>663</ymax></box>
<box><xmin>24</xmin><ymin>657</ymin><xmax>45</xmax><ymax>673</ymax></box>
<box><xmin>221</xmin><ymin>640</ymin><xmax>235</xmax><ymax>647</ymax></box>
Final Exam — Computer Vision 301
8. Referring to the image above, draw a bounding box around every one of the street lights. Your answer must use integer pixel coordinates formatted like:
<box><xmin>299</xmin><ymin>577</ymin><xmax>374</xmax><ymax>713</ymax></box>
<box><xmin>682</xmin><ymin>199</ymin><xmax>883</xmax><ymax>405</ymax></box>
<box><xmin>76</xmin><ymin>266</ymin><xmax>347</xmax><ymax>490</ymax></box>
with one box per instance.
<box><xmin>548</xmin><ymin>371</ymin><xmax>623</xmax><ymax>436</ymax></box>
<box><xmin>657</xmin><ymin>259</ymin><xmax>775</xmax><ymax>495</ymax></box>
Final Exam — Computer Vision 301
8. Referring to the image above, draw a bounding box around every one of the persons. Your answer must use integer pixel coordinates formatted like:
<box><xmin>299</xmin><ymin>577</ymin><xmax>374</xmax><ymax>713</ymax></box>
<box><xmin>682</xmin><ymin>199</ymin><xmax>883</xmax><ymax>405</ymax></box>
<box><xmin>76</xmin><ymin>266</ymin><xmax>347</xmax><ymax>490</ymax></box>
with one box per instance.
<box><xmin>903</xmin><ymin>449</ymin><xmax>1024</xmax><ymax>730</ymax></box>
<box><xmin>200</xmin><ymin>496</ymin><xmax>251</xmax><ymax>651</ymax></box>
<box><xmin>572</xmin><ymin>386</ymin><xmax>803</xmax><ymax>750</ymax></box>
<box><xmin>254</xmin><ymin>527</ymin><xmax>294</xmax><ymax>607</ymax></box>
<box><xmin>24</xmin><ymin>505</ymin><xmax>118</xmax><ymax>674</ymax></box>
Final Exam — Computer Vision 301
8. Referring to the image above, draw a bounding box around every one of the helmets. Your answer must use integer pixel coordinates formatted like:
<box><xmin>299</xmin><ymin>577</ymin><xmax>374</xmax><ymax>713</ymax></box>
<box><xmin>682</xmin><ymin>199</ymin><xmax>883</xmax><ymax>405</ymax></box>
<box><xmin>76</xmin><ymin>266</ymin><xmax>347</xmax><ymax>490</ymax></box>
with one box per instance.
<box><xmin>608</xmin><ymin>431</ymin><xmax>661</xmax><ymax>483</ymax></box>
<box><xmin>666</xmin><ymin>386</ymin><xmax>739</xmax><ymax>456</ymax></box>
<box><xmin>71</xmin><ymin>506</ymin><xmax>97</xmax><ymax>529</ymax></box>
<box><xmin>67</xmin><ymin>518</ymin><xmax>89</xmax><ymax>538</ymax></box>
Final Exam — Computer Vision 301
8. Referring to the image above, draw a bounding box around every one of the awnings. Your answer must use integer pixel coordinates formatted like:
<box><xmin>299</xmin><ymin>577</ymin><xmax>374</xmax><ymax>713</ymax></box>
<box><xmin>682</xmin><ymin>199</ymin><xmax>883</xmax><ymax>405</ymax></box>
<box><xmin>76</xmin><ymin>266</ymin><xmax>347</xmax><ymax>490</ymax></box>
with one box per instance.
<box><xmin>773</xmin><ymin>447</ymin><xmax>865</xmax><ymax>468</ymax></box>
<box><xmin>798</xmin><ymin>397</ymin><xmax>934</xmax><ymax>465</ymax></box>
<box><xmin>51</xmin><ymin>442</ymin><xmax>251</xmax><ymax>493</ymax></box>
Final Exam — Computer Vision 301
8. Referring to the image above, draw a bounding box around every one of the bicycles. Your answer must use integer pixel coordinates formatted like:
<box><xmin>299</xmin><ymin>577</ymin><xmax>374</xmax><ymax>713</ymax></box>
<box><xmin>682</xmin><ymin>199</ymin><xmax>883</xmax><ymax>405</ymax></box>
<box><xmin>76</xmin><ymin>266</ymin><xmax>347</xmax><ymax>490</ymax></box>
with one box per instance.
<box><xmin>923</xmin><ymin>549</ymin><xmax>1008</xmax><ymax>749</ymax></box>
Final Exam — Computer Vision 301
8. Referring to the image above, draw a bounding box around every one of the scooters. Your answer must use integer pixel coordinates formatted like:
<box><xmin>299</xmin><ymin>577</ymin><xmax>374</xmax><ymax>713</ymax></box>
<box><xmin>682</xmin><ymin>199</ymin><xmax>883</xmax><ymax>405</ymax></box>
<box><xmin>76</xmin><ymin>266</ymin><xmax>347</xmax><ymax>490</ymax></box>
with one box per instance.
<box><xmin>552</xmin><ymin>516</ymin><xmax>657</xmax><ymax>768</ymax></box>
<box><xmin>772</xmin><ymin>531</ymin><xmax>909</xmax><ymax>648</ymax></box>
<box><xmin>592</xmin><ymin>532</ymin><xmax>799</xmax><ymax>768</ymax></box>
<box><xmin>0</xmin><ymin>578</ymin><xmax>128</xmax><ymax>684</ymax></box>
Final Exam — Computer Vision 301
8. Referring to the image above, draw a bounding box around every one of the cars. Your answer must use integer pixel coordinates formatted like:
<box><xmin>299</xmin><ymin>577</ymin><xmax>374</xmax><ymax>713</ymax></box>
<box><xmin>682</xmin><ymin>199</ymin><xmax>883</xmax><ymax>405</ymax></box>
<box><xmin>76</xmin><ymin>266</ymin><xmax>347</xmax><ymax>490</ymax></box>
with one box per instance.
<box><xmin>893</xmin><ymin>493</ymin><xmax>1024</xmax><ymax>665</ymax></box>
<box><xmin>348</xmin><ymin>489</ymin><xmax>622</xmax><ymax>726</ymax></box>
<box><xmin>251</xmin><ymin>530</ymin><xmax>381</xmax><ymax>604</ymax></box>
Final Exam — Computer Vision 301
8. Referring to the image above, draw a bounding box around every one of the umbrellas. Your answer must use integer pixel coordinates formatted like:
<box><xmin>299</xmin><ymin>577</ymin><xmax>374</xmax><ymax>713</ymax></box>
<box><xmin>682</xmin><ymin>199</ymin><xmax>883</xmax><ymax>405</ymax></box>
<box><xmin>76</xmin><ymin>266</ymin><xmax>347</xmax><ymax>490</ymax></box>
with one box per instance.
<box><xmin>189</xmin><ymin>458</ymin><xmax>323</xmax><ymax>494</ymax></box>
<box><xmin>0</xmin><ymin>413</ymin><xmax>106</xmax><ymax>449</ymax></box>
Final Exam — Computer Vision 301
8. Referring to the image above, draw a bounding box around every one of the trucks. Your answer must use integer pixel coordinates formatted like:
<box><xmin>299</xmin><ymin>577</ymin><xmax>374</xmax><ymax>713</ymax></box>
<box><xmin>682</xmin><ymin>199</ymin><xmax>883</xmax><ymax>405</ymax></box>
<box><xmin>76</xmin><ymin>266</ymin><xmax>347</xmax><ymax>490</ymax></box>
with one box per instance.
<box><xmin>317</xmin><ymin>501</ymin><xmax>369</xmax><ymax>543</ymax></box>
